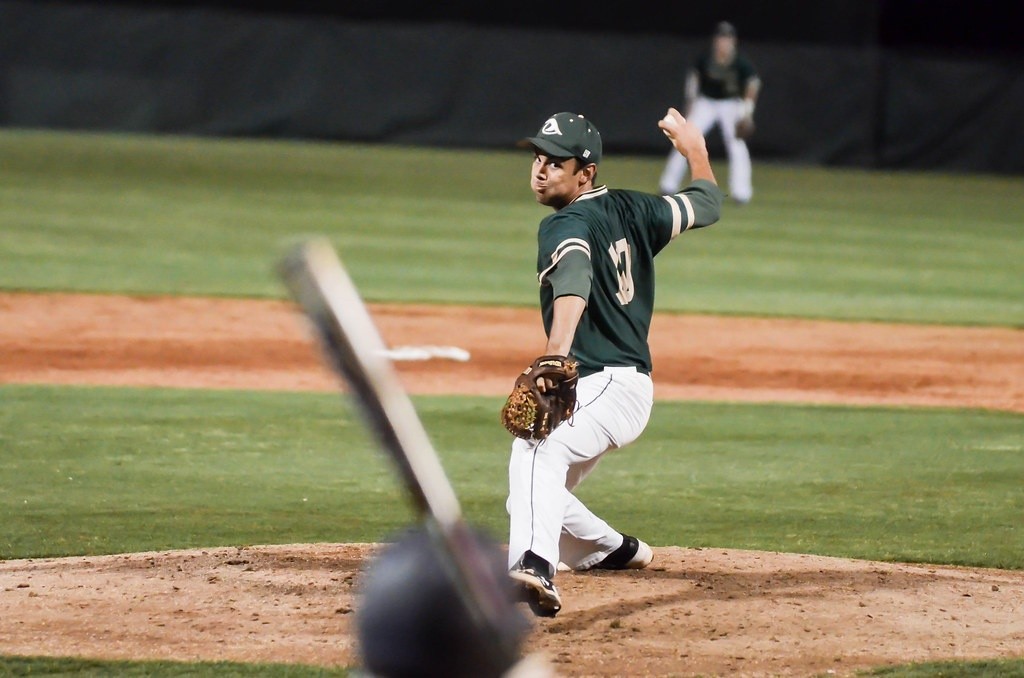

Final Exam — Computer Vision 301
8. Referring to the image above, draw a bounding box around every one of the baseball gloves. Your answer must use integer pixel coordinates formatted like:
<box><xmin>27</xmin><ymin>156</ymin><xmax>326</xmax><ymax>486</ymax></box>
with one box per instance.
<box><xmin>735</xmin><ymin>115</ymin><xmax>756</xmax><ymax>138</ymax></box>
<box><xmin>503</xmin><ymin>355</ymin><xmax>580</xmax><ymax>439</ymax></box>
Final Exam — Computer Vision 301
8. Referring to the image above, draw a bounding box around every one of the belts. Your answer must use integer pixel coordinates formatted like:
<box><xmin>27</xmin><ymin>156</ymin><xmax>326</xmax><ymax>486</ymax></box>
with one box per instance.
<box><xmin>577</xmin><ymin>365</ymin><xmax>649</xmax><ymax>378</ymax></box>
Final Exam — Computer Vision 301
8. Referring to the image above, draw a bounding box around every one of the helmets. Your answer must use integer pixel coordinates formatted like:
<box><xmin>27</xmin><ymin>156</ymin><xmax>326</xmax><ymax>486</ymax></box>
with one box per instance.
<box><xmin>351</xmin><ymin>524</ymin><xmax>531</xmax><ymax>678</ymax></box>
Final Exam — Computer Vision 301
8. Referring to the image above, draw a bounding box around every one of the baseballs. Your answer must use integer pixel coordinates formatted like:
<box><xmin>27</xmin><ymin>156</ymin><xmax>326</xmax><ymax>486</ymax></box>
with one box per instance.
<box><xmin>662</xmin><ymin>115</ymin><xmax>686</xmax><ymax>136</ymax></box>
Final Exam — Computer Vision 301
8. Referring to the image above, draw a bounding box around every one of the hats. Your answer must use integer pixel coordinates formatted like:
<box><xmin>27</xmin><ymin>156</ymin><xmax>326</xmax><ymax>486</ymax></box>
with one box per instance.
<box><xmin>525</xmin><ymin>112</ymin><xmax>603</xmax><ymax>166</ymax></box>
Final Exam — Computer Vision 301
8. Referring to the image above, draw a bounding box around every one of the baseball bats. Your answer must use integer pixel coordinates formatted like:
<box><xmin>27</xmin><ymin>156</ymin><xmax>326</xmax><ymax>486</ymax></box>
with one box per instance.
<box><xmin>272</xmin><ymin>234</ymin><xmax>553</xmax><ymax>678</ymax></box>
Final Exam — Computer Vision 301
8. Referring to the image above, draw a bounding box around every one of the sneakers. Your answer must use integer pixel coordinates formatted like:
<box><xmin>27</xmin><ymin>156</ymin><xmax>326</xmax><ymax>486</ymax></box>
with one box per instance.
<box><xmin>591</xmin><ymin>533</ymin><xmax>655</xmax><ymax>569</ymax></box>
<box><xmin>504</xmin><ymin>565</ymin><xmax>562</xmax><ymax>615</ymax></box>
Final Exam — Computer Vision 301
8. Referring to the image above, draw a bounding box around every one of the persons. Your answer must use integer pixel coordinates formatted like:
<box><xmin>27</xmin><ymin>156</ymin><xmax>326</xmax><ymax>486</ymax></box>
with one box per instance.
<box><xmin>660</xmin><ymin>24</ymin><xmax>763</xmax><ymax>204</ymax></box>
<box><xmin>501</xmin><ymin>108</ymin><xmax>721</xmax><ymax>618</ymax></box>
<box><xmin>354</xmin><ymin>521</ymin><xmax>551</xmax><ymax>678</ymax></box>
